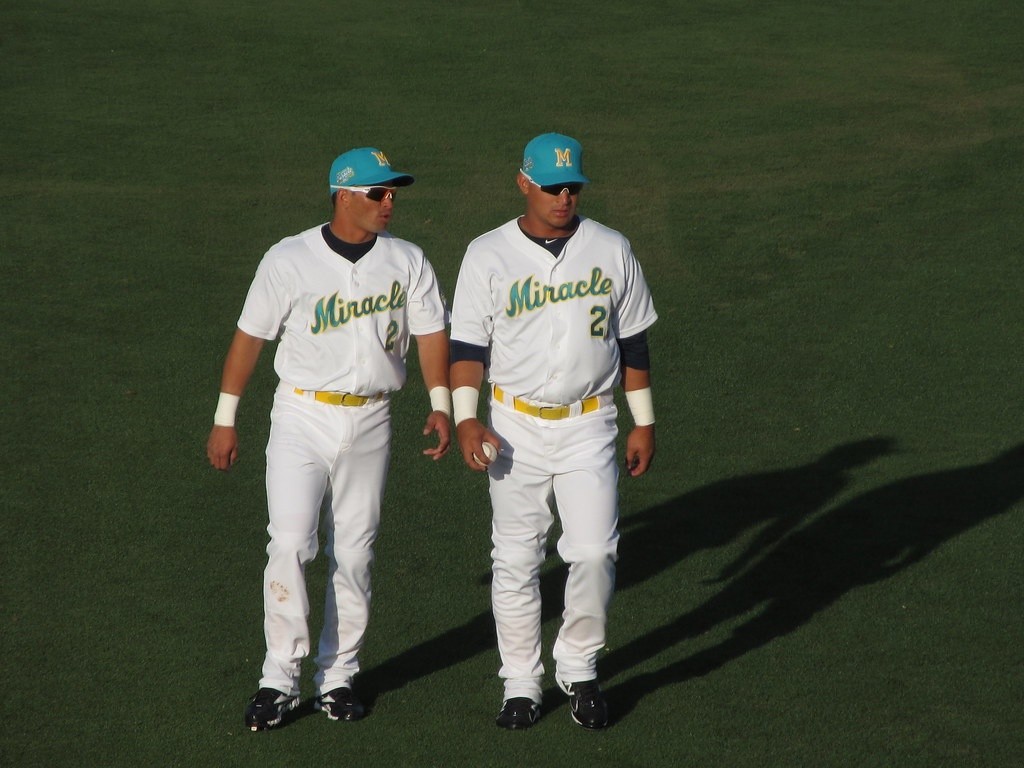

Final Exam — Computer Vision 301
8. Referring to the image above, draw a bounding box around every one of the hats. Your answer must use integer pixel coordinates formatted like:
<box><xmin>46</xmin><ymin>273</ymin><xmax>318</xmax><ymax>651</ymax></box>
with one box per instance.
<box><xmin>329</xmin><ymin>146</ymin><xmax>414</xmax><ymax>198</ymax></box>
<box><xmin>522</xmin><ymin>133</ymin><xmax>593</xmax><ymax>187</ymax></box>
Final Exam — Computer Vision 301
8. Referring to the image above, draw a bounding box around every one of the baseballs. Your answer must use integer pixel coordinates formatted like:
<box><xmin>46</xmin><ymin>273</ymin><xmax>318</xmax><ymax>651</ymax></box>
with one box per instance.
<box><xmin>471</xmin><ymin>441</ymin><xmax>500</xmax><ymax>469</ymax></box>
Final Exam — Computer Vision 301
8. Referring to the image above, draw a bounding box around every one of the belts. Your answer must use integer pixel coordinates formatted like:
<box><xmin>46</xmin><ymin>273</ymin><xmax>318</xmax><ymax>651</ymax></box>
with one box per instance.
<box><xmin>494</xmin><ymin>385</ymin><xmax>601</xmax><ymax>420</ymax></box>
<box><xmin>295</xmin><ymin>388</ymin><xmax>384</xmax><ymax>407</ymax></box>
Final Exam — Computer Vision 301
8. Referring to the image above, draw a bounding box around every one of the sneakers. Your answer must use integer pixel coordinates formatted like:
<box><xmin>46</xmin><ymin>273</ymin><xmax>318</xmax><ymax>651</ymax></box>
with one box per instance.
<box><xmin>495</xmin><ymin>697</ymin><xmax>540</xmax><ymax>731</ymax></box>
<box><xmin>556</xmin><ymin>673</ymin><xmax>609</xmax><ymax>732</ymax></box>
<box><xmin>244</xmin><ymin>688</ymin><xmax>300</xmax><ymax>731</ymax></box>
<box><xmin>315</xmin><ymin>686</ymin><xmax>352</xmax><ymax>722</ymax></box>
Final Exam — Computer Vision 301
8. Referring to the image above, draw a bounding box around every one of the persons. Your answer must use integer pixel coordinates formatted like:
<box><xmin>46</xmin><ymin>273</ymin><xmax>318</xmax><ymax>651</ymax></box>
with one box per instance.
<box><xmin>450</xmin><ymin>132</ymin><xmax>659</xmax><ymax>729</ymax></box>
<box><xmin>206</xmin><ymin>147</ymin><xmax>450</xmax><ymax>732</ymax></box>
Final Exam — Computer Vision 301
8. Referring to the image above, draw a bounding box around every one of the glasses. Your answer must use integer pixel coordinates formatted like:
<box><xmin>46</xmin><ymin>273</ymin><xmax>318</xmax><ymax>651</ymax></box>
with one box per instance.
<box><xmin>520</xmin><ymin>168</ymin><xmax>584</xmax><ymax>196</ymax></box>
<box><xmin>330</xmin><ymin>183</ymin><xmax>397</xmax><ymax>203</ymax></box>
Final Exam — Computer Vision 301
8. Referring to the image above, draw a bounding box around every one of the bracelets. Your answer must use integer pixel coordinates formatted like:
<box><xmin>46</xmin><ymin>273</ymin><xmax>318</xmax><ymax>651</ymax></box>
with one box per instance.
<box><xmin>624</xmin><ymin>386</ymin><xmax>656</xmax><ymax>426</ymax></box>
<box><xmin>452</xmin><ymin>386</ymin><xmax>479</xmax><ymax>427</ymax></box>
<box><xmin>213</xmin><ymin>392</ymin><xmax>240</xmax><ymax>427</ymax></box>
<box><xmin>429</xmin><ymin>386</ymin><xmax>450</xmax><ymax>417</ymax></box>
